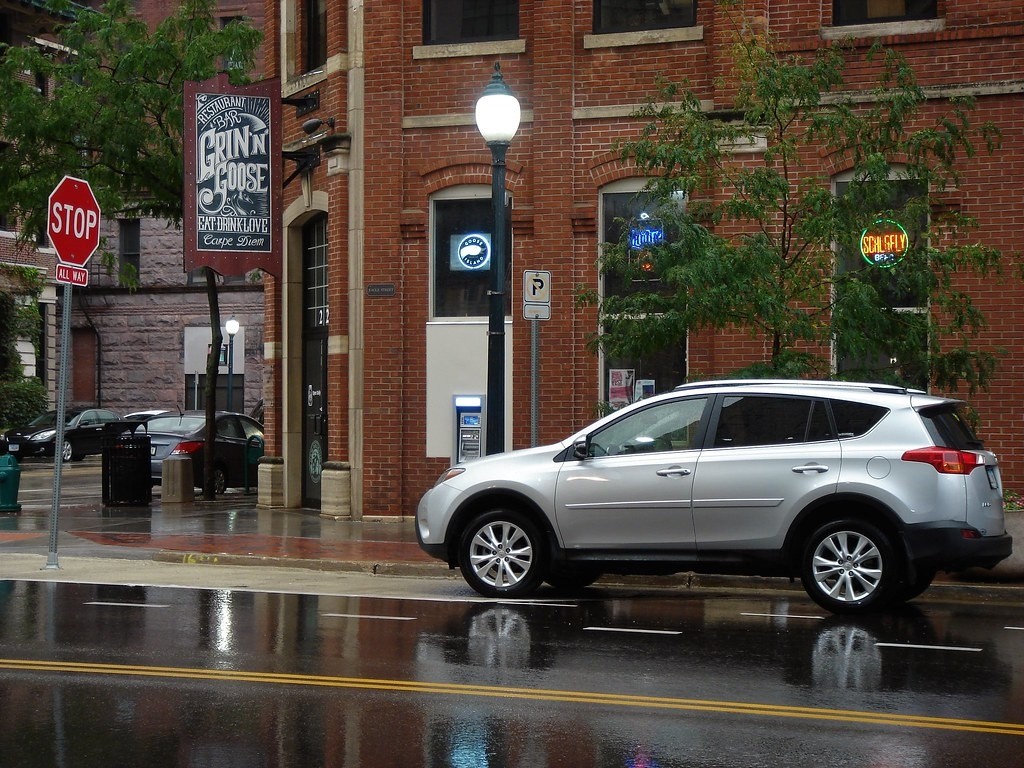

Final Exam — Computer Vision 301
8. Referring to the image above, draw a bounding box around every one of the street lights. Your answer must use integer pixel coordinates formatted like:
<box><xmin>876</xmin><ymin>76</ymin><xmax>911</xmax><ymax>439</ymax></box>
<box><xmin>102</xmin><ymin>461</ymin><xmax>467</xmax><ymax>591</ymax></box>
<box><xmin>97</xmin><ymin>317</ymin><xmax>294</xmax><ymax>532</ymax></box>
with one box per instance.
<box><xmin>226</xmin><ymin>312</ymin><xmax>240</xmax><ymax>412</ymax></box>
<box><xmin>474</xmin><ymin>61</ymin><xmax>522</xmax><ymax>456</ymax></box>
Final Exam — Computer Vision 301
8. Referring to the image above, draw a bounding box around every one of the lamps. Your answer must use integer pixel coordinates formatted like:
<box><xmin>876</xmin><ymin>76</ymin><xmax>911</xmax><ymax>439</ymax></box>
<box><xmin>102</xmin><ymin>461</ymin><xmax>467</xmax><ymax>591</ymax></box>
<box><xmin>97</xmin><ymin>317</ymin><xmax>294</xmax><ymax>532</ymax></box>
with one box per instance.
<box><xmin>302</xmin><ymin>117</ymin><xmax>335</xmax><ymax>135</ymax></box>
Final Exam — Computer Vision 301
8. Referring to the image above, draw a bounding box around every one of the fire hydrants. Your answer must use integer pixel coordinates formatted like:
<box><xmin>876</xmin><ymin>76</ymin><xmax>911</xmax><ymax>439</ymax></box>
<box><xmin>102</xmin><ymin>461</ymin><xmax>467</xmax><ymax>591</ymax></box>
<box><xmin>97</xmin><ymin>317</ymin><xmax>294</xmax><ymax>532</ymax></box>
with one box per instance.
<box><xmin>0</xmin><ymin>450</ymin><xmax>23</xmax><ymax>512</ymax></box>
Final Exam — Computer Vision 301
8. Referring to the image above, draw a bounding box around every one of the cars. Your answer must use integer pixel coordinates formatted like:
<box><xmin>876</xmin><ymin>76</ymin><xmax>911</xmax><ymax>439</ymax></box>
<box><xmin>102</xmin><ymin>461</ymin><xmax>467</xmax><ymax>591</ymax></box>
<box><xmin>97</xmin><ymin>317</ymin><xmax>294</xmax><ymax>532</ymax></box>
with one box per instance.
<box><xmin>5</xmin><ymin>406</ymin><xmax>129</xmax><ymax>464</ymax></box>
<box><xmin>115</xmin><ymin>399</ymin><xmax>264</xmax><ymax>494</ymax></box>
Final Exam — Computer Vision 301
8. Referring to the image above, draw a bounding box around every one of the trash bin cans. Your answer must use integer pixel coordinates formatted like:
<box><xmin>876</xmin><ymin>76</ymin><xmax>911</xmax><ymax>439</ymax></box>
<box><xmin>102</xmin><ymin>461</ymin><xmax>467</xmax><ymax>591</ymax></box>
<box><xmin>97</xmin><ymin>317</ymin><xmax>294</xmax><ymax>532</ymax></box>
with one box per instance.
<box><xmin>102</xmin><ymin>421</ymin><xmax>153</xmax><ymax>508</ymax></box>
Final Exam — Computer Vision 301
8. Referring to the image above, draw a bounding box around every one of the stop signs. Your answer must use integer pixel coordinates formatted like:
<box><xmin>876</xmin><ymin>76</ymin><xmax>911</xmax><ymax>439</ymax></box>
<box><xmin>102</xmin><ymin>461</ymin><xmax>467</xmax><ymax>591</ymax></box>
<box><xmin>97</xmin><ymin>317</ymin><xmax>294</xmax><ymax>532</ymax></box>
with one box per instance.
<box><xmin>46</xmin><ymin>176</ymin><xmax>101</xmax><ymax>267</ymax></box>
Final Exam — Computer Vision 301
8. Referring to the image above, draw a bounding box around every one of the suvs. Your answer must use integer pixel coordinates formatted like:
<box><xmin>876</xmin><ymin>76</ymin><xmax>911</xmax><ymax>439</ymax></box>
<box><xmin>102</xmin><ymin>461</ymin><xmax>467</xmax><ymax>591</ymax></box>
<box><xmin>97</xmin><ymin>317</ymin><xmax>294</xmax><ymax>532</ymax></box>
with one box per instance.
<box><xmin>414</xmin><ymin>378</ymin><xmax>1013</xmax><ymax>617</ymax></box>
